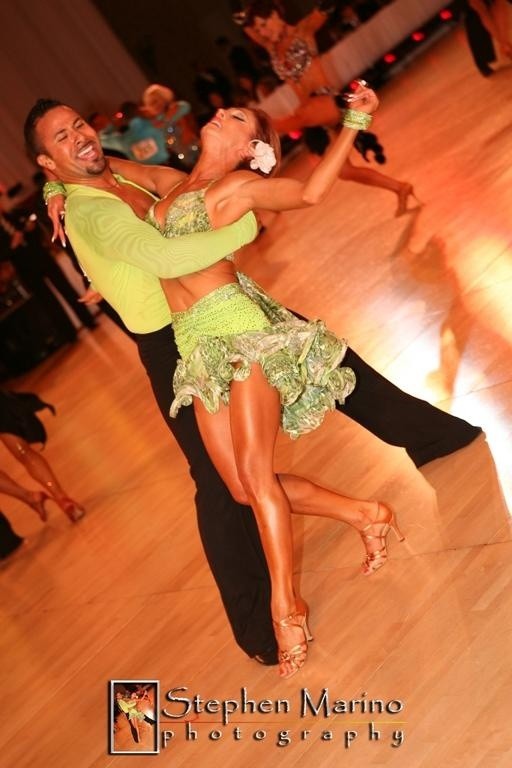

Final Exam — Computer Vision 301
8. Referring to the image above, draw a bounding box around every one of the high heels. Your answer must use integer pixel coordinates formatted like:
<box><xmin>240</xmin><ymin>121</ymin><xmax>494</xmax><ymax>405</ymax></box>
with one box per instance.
<box><xmin>254</xmin><ymin>599</ymin><xmax>313</xmax><ymax>680</ymax></box>
<box><xmin>394</xmin><ymin>182</ymin><xmax>416</xmax><ymax>217</ymax></box>
<box><xmin>30</xmin><ymin>491</ymin><xmax>85</xmax><ymax>522</ymax></box>
<box><xmin>359</xmin><ymin>500</ymin><xmax>405</xmax><ymax>576</ymax></box>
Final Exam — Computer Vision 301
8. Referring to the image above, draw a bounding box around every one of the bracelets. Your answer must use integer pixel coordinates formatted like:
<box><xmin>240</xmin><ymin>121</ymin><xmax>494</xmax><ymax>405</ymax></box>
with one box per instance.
<box><xmin>42</xmin><ymin>183</ymin><xmax>64</xmax><ymax>201</ymax></box>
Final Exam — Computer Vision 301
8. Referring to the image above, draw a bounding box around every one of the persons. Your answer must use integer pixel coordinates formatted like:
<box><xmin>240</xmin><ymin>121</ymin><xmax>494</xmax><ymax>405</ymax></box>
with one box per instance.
<box><xmin>0</xmin><ymin>514</ymin><xmax>25</xmax><ymax>558</ymax></box>
<box><xmin>242</xmin><ymin>7</ymin><xmax>425</xmax><ymax>218</ymax></box>
<box><xmin>1</xmin><ymin>388</ymin><xmax>84</xmax><ymax>523</ymax></box>
<box><xmin>1</xmin><ymin>169</ymin><xmax>98</xmax><ymax>343</ymax></box>
<box><xmin>451</xmin><ymin>0</ymin><xmax>512</xmax><ymax>76</ymax></box>
